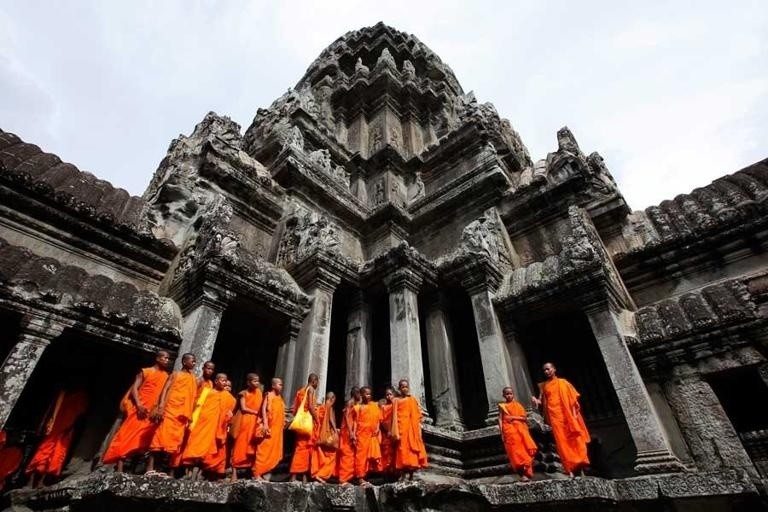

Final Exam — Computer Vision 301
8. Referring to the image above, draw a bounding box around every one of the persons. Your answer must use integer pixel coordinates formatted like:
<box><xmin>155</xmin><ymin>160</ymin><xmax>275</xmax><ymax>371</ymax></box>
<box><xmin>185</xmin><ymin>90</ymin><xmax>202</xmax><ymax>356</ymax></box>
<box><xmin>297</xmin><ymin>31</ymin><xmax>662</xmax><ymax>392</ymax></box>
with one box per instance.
<box><xmin>497</xmin><ymin>387</ymin><xmax>538</xmax><ymax>482</ymax></box>
<box><xmin>22</xmin><ymin>382</ymin><xmax>91</xmax><ymax>489</ymax></box>
<box><xmin>531</xmin><ymin>363</ymin><xmax>592</xmax><ymax>478</ymax></box>
<box><xmin>0</xmin><ymin>430</ymin><xmax>24</xmax><ymax>492</ymax></box>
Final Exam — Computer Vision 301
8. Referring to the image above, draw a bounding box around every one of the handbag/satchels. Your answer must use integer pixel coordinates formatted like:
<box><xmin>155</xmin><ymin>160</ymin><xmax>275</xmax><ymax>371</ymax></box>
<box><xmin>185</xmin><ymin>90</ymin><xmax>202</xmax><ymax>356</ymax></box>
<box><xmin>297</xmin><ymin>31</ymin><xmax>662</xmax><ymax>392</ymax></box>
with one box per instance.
<box><xmin>119</xmin><ymin>394</ymin><xmax>138</xmax><ymax>416</ymax></box>
<box><xmin>389</xmin><ymin>419</ymin><xmax>399</xmax><ymax>442</ymax></box>
<box><xmin>36</xmin><ymin>414</ymin><xmax>56</xmax><ymax>436</ymax></box>
<box><xmin>150</xmin><ymin>403</ymin><xmax>160</xmax><ymax>424</ymax></box>
<box><xmin>315</xmin><ymin>422</ymin><xmax>341</xmax><ymax>449</ymax></box>
<box><xmin>287</xmin><ymin>404</ymin><xmax>316</xmax><ymax>437</ymax></box>
<box><xmin>229</xmin><ymin>414</ymin><xmax>243</xmax><ymax>439</ymax></box>
<box><xmin>255</xmin><ymin>413</ymin><xmax>273</xmax><ymax>438</ymax></box>
